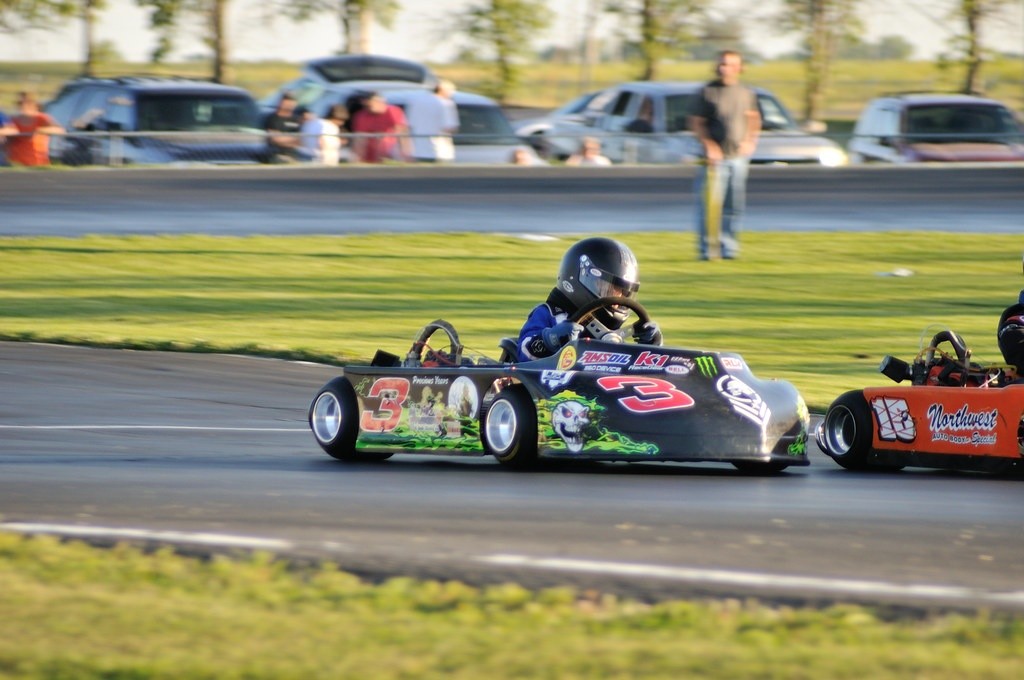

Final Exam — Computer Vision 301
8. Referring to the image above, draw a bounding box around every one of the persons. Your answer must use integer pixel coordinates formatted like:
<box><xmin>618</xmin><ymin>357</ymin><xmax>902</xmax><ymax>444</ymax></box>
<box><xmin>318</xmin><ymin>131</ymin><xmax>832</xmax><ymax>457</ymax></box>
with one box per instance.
<box><xmin>516</xmin><ymin>236</ymin><xmax>663</xmax><ymax>363</ymax></box>
<box><xmin>511</xmin><ymin>148</ymin><xmax>529</xmax><ymax>165</ymax></box>
<box><xmin>566</xmin><ymin>138</ymin><xmax>611</xmax><ymax>166</ymax></box>
<box><xmin>687</xmin><ymin>51</ymin><xmax>762</xmax><ymax>260</ymax></box>
<box><xmin>0</xmin><ymin>109</ymin><xmax>20</xmax><ymax>167</ymax></box>
<box><xmin>409</xmin><ymin>81</ymin><xmax>458</xmax><ymax>162</ymax></box>
<box><xmin>294</xmin><ymin>104</ymin><xmax>350</xmax><ymax>163</ymax></box>
<box><xmin>262</xmin><ymin>91</ymin><xmax>314</xmax><ymax>163</ymax></box>
<box><xmin>353</xmin><ymin>92</ymin><xmax>411</xmax><ymax>163</ymax></box>
<box><xmin>5</xmin><ymin>91</ymin><xmax>66</xmax><ymax>166</ymax></box>
<box><xmin>997</xmin><ymin>302</ymin><xmax>1024</xmax><ymax>376</ymax></box>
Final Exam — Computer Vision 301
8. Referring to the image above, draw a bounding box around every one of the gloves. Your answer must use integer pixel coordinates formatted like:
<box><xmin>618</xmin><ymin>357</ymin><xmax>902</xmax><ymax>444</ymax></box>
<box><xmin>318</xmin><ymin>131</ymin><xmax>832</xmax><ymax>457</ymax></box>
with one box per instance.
<box><xmin>542</xmin><ymin>320</ymin><xmax>584</xmax><ymax>354</ymax></box>
<box><xmin>632</xmin><ymin>319</ymin><xmax>664</xmax><ymax>346</ymax></box>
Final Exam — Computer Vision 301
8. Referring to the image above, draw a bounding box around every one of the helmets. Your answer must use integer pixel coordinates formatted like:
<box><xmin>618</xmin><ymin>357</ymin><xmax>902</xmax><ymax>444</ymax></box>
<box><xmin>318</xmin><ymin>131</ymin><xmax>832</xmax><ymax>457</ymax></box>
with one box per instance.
<box><xmin>557</xmin><ymin>237</ymin><xmax>640</xmax><ymax>330</ymax></box>
<box><xmin>997</xmin><ymin>303</ymin><xmax>1024</xmax><ymax>377</ymax></box>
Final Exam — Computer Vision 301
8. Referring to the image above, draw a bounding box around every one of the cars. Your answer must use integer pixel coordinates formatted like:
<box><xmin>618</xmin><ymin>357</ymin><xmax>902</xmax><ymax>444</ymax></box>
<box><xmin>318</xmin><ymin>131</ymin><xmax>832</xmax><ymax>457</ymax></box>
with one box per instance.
<box><xmin>39</xmin><ymin>54</ymin><xmax>1024</xmax><ymax>165</ymax></box>
